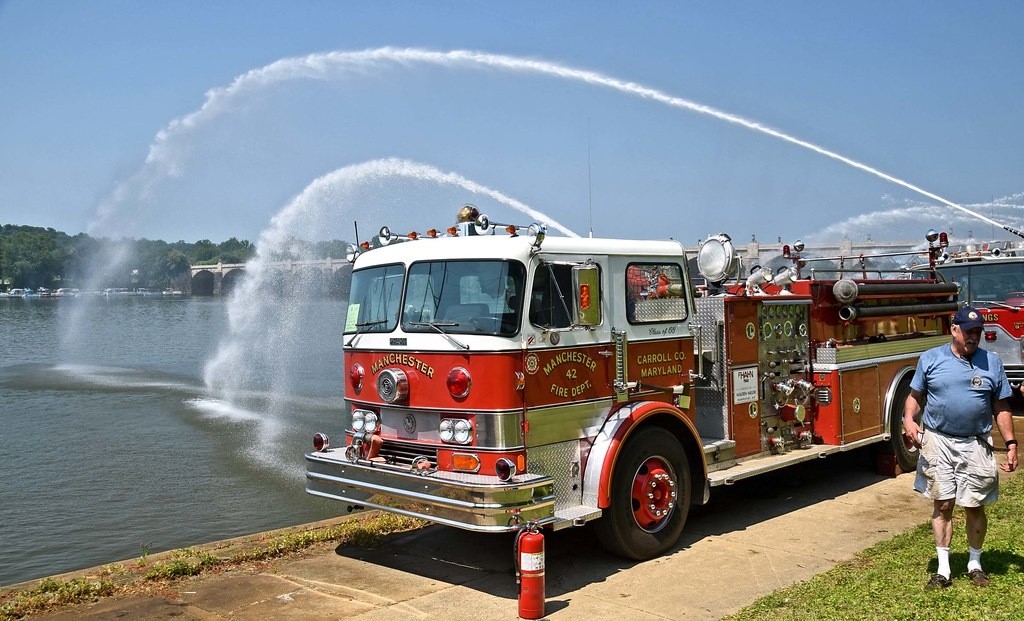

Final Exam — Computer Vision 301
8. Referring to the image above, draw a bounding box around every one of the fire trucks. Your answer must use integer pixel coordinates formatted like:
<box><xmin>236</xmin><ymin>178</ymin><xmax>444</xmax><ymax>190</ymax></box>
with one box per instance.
<box><xmin>304</xmin><ymin>203</ymin><xmax>967</xmax><ymax>562</ymax></box>
<box><xmin>896</xmin><ymin>226</ymin><xmax>1024</xmax><ymax>393</ymax></box>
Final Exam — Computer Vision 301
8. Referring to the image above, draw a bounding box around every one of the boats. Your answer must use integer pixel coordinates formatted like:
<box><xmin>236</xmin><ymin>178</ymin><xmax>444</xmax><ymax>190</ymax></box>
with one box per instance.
<box><xmin>0</xmin><ymin>287</ymin><xmax>182</xmax><ymax>298</ymax></box>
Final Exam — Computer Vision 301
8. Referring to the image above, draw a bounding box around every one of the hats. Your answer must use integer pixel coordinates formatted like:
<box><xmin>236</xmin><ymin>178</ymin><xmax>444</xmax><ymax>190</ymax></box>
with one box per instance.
<box><xmin>952</xmin><ymin>306</ymin><xmax>985</xmax><ymax>331</ymax></box>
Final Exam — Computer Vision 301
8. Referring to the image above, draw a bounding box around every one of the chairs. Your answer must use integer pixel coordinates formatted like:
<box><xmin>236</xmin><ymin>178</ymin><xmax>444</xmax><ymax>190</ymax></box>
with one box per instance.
<box><xmin>442</xmin><ymin>295</ymin><xmax>543</xmax><ymax>331</ymax></box>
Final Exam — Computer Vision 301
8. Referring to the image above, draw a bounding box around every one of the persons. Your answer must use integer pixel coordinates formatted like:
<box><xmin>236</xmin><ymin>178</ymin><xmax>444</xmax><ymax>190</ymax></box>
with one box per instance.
<box><xmin>904</xmin><ymin>306</ymin><xmax>1019</xmax><ymax>592</ymax></box>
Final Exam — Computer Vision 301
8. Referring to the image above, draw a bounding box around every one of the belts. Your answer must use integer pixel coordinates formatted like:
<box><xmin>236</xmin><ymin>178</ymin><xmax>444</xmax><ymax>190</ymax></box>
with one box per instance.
<box><xmin>939</xmin><ymin>429</ymin><xmax>1010</xmax><ymax>451</ymax></box>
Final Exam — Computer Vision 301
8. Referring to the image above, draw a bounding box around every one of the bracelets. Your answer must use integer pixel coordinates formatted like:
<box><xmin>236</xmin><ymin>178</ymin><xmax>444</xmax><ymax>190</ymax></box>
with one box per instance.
<box><xmin>1006</xmin><ymin>440</ymin><xmax>1018</xmax><ymax>448</ymax></box>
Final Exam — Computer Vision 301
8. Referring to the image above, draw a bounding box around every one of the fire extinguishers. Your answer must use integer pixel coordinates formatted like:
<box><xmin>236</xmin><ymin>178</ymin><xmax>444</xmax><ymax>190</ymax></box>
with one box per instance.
<box><xmin>517</xmin><ymin>520</ymin><xmax>546</xmax><ymax>619</ymax></box>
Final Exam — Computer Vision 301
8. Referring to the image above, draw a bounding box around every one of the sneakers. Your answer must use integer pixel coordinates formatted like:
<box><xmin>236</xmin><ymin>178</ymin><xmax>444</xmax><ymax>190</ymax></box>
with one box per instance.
<box><xmin>930</xmin><ymin>573</ymin><xmax>953</xmax><ymax>587</ymax></box>
<box><xmin>965</xmin><ymin>569</ymin><xmax>990</xmax><ymax>586</ymax></box>
